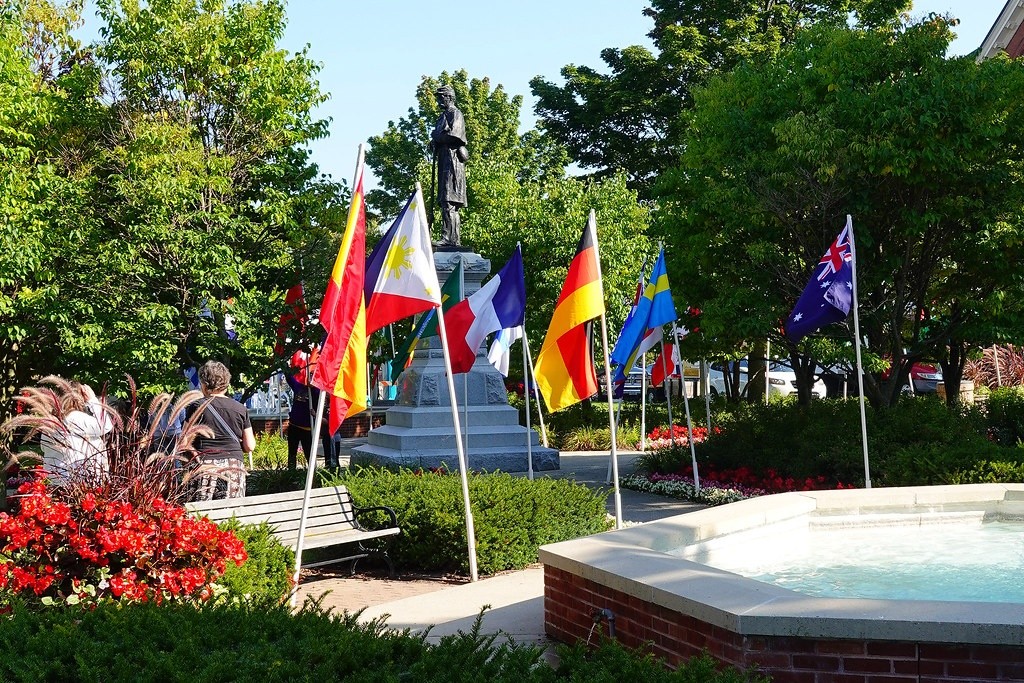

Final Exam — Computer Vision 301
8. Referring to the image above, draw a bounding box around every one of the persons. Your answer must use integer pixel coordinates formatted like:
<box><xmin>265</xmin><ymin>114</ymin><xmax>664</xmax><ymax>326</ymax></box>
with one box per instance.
<box><xmin>426</xmin><ymin>86</ymin><xmax>470</xmax><ymax>247</ymax></box>
<box><xmin>279</xmin><ymin>361</ymin><xmax>343</xmax><ymax>481</ymax></box>
<box><xmin>149</xmin><ymin>357</ymin><xmax>256</xmax><ymax>508</ymax></box>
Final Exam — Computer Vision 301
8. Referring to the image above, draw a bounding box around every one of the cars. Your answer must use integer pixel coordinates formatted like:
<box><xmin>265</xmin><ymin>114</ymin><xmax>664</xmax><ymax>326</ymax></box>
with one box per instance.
<box><xmin>622</xmin><ymin>362</ymin><xmax>679</xmax><ymax>402</ymax></box>
<box><xmin>879</xmin><ymin>348</ymin><xmax>944</xmax><ymax>397</ymax></box>
<box><xmin>709</xmin><ymin>351</ymin><xmax>827</xmax><ymax>410</ymax></box>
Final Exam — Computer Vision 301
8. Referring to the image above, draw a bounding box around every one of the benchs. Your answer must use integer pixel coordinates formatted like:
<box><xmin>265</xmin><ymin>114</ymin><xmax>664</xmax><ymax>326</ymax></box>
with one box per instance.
<box><xmin>180</xmin><ymin>484</ymin><xmax>400</xmax><ymax>580</ymax></box>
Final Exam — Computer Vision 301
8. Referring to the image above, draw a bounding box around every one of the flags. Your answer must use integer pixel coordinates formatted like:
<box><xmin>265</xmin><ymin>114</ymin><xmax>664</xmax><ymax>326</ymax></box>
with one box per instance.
<box><xmin>783</xmin><ymin>215</ymin><xmax>857</xmax><ymax>345</ymax></box>
<box><xmin>273</xmin><ymin>144</ymin><xmax>688</xmax><ymax>437</ymax></box>
